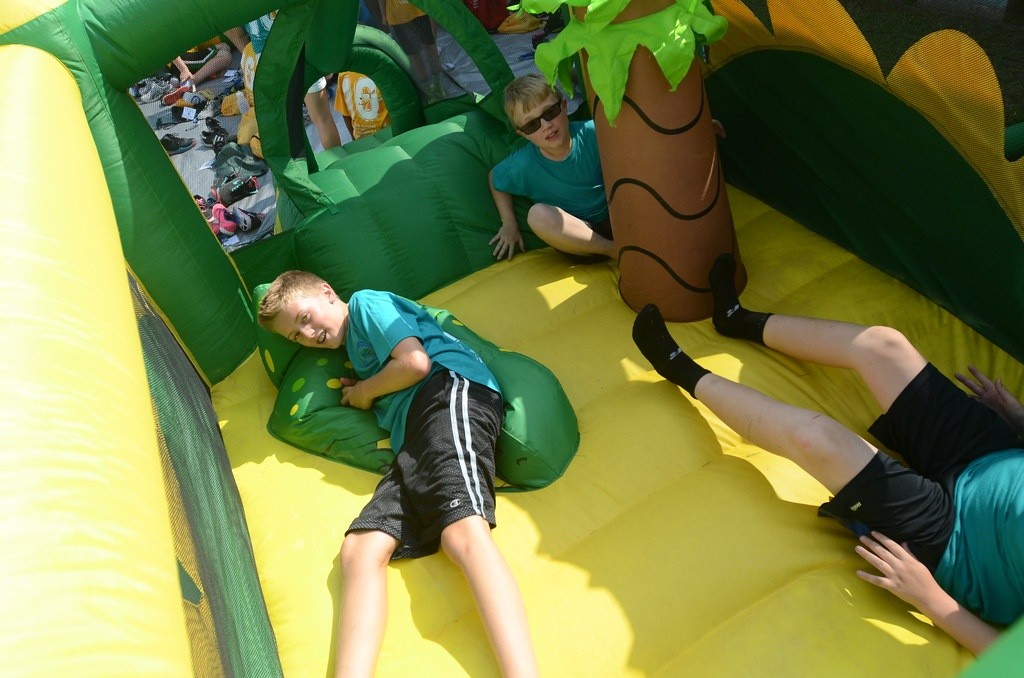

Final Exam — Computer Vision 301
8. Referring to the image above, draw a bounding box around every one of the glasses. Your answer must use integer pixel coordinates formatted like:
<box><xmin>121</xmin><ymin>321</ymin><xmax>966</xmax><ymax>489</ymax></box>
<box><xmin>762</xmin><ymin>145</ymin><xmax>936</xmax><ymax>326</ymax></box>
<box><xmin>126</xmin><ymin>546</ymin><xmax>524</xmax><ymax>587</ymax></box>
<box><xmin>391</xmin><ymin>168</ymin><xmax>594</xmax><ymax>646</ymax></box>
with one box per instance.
<box><xmin>516</xmin><ymin>97</ymin><xmax>562</xmax><ymax>135</ymax></box>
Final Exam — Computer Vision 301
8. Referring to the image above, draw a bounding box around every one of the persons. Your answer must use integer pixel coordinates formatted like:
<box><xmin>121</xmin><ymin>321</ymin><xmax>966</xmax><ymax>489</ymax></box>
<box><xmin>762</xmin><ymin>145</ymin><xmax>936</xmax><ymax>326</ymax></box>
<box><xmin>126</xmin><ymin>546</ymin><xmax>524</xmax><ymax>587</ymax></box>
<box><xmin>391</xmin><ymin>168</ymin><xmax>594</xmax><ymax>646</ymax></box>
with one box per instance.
<box><xmin>632</xmin><ymin>253</ymin><xmax>1024</xmax><ymax>659</ymax></box>
<box><xmin>490</xmin><ymin>74</ymin><xmax>619</xmax><ymax>260</ymax></box>
<box><xmin>167</xmin><ymin>36</ymin><xmax>232</xmax><ymax>86</ymax></box>
<box><xmin>334</xmin><ymin>71</ymin><xmax>391</xmax><ymax>140</ymax></box>
<box><xmin>259</xmin><ymin>270</ymin><xmax>537</xmax><ymax>677</ymax></box>
<box><xmin>224</xmin><ymin>8</ymin><xmax>342</xmax><ymax>150</ymax></box>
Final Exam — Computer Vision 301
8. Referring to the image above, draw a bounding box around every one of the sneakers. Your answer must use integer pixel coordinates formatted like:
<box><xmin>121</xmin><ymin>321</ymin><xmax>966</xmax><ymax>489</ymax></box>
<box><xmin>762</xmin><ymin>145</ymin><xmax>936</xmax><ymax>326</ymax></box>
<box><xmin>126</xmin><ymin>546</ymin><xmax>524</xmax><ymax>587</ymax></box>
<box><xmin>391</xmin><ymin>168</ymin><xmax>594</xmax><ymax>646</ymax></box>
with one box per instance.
<box><xmin>128</xmin><ymin>67</ymin><xmax>269</xmax><ymax>236</ymax></box>
